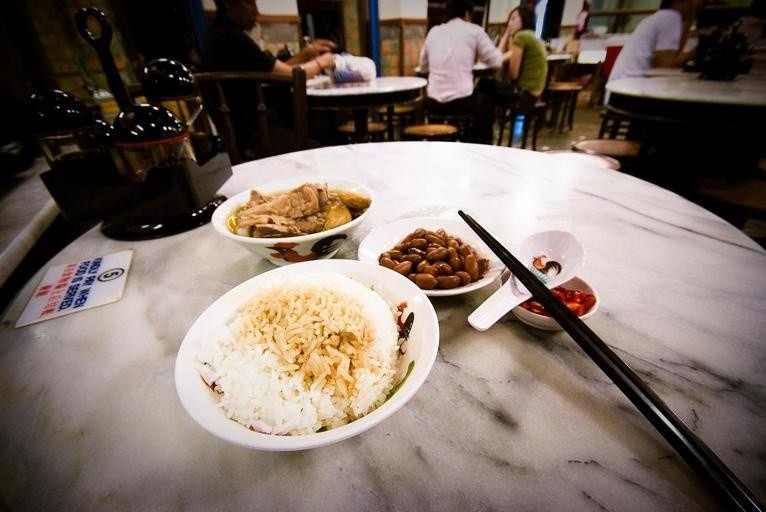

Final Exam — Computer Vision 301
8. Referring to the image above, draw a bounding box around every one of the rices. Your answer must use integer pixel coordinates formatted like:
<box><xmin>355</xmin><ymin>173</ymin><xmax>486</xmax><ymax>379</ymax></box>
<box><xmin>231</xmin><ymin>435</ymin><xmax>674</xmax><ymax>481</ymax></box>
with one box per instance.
<box><xmin>199</xmin><ymin>275</ymin><xmax>399</xmax><ymax>436</ymax></box>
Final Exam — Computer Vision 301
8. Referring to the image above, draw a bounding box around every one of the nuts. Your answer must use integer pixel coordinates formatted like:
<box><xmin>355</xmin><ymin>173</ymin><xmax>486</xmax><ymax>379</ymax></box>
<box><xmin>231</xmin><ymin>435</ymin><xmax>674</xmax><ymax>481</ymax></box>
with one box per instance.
<box><xmin>379</xmin><ymin>226</ymin><xmax>490</xmax><ymax>289</ymax></box>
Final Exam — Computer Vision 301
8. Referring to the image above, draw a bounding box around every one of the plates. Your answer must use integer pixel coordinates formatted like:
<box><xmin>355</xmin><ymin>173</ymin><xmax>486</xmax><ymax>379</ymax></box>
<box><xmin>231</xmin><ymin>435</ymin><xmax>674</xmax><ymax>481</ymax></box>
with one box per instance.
<box><xmin>500</xmin><ymin>270</ymin><xmax>598</xmax><ymax>330</ymax></box>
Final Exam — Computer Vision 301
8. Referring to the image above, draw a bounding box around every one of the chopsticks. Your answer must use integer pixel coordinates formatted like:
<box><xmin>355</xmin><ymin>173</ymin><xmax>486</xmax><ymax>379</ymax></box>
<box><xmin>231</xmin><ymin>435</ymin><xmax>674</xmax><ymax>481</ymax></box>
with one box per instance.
<box><xmin>457</xmin><ymin>210</ymin><xmax>766</xmax><ymax>512</ymax></box>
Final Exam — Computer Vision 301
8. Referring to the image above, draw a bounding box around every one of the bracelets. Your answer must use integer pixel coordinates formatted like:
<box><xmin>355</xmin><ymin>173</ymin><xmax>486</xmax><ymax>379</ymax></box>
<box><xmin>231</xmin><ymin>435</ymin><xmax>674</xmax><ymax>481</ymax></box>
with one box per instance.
<box><xmin>314</xmin><ymin>57</ymin><xmax>325</xmax><ymax>74</ymax></box>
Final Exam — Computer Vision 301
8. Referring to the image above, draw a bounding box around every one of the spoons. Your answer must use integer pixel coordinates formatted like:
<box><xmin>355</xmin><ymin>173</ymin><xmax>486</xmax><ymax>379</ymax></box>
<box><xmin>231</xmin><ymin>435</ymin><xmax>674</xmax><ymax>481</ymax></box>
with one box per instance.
<box><xmin>468</xmin><ymin>231</ymin><xmax>587</xmax><ymax>332</ymax></box>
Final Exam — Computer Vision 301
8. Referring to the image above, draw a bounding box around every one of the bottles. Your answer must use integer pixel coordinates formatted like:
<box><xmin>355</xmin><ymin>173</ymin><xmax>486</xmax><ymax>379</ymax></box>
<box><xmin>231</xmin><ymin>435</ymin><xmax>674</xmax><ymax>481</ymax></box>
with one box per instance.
<box><xmin>112</xmin><ymin>103</ymin><xmax>197</xmax><ymax>184</ymax></box>
<box><xmin>144</xmin><ymin>58</ymin><xmax>218</xmax><ymax>157</ymax></box>
<box><xmin>26</xmin><ymin>89</ymin><xmax>100</xmax><ymax>174</ymax></box>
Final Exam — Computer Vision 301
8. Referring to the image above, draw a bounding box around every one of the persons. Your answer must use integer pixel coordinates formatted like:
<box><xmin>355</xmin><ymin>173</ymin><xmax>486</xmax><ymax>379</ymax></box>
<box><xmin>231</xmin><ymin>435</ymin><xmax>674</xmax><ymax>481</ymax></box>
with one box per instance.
<box><xmin>602</xmin><ymin>0</ymin><xmax>709</xmax><ymax>105</ymax></box>
<box><xmin>477</xmin><ymin>6</ymin><xmax>549</xmax><ymax>103</ymax></box>
<box><xmin>197</xmin><ymin>0</ymin><xmax>338</xmax><ymax>150</ymax></box>
<box><xmin>412</xmin><ymin>1</ymin><xmax>505</xmax><ymax>144</ymax></box>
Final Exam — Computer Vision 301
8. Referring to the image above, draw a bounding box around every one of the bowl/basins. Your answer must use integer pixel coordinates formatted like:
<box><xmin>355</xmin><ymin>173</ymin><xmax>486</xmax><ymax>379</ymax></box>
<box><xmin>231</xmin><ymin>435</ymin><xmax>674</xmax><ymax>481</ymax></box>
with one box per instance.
<box><xmin>174</xmin><ymin>260</ymin><xmax>439</xmax><ymax>454</ymax></box>
<box><xmin>211</xmin><ymin>181</ymin><xmax>371</xmax><ymax>266</ymax></box>
<box><xmin>357</xmin><ymin>217</ymin><xmax>506</xmax><ymax>297</ymax></box>
